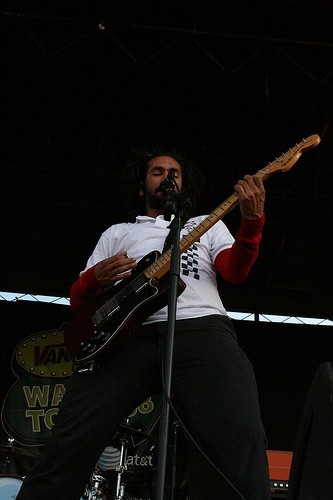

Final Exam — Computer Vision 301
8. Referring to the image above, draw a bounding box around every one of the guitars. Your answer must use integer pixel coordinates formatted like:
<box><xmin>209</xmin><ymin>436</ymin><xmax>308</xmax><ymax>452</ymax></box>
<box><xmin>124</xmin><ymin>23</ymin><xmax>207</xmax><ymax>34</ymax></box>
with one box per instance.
<box><xmin>62</xmin><ymin>132</ymin><xmax>324</xmax><ymax>373</ymax></box>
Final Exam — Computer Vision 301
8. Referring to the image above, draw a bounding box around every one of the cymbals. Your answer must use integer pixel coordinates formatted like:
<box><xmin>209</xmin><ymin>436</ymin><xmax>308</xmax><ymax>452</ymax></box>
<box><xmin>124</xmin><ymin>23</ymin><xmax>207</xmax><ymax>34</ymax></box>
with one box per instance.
<box><xmin>0</xmin><ymin>438</ymin><xmax>35</xmax><ymax>457</ymax></box>
<box><xmin>118</xmin><ymin>422</ymin><xmax>147</xmax><ymax>438</ymax></box>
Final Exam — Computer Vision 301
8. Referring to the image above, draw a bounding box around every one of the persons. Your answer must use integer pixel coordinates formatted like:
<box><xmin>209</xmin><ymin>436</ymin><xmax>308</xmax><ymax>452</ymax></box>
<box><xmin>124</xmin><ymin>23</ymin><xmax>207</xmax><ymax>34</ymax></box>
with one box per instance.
<box><xmin>15</xmin><ymin>151</ymin><xmax>271</xmax><ymax>500</ymax></box>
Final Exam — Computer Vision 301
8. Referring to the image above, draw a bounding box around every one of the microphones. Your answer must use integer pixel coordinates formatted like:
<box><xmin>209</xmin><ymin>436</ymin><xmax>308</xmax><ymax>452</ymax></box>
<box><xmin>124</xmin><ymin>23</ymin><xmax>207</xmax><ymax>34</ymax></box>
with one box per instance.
<box><xmin>159</xmin><ymin>168</ymin><xmax>176</xmax><ymax>195</ymax></box>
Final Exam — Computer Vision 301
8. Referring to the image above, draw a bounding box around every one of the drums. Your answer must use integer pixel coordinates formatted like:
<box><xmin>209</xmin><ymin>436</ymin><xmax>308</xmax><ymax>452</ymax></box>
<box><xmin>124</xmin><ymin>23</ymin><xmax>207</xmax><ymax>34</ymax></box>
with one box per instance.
<box><xmin>0</xmin><ymin>472</ymin><xmax>27</xmax><ymax>500</ymax></box>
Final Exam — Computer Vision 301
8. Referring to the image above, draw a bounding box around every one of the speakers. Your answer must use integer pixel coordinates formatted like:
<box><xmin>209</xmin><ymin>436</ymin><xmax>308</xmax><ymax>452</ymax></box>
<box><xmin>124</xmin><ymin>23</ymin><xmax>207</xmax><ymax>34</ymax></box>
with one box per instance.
<box><xmin>285</xmin><ymin>362</ymin><xmax>333</xmax><ymax>500</ymax></box>
